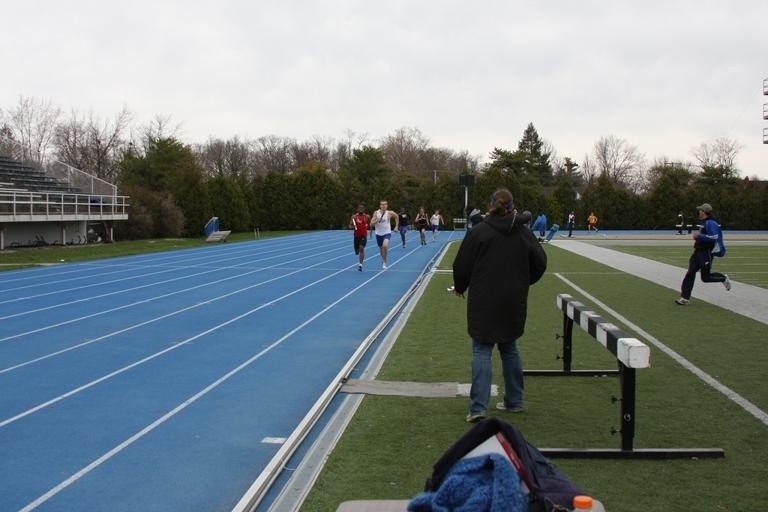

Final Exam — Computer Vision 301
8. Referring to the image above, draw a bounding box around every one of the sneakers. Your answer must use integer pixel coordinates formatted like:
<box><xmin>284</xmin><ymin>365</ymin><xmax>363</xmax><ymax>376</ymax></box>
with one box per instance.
<box><xmin>721</xmin><ymin>273</ymin><xmax>731</xmax><ymax>291</ymax></box>
<box><xmin>674</xmin><ymin>297</ymin><xmax>692</xmax><ymax>306</ymax></box>
<box><xmin>495</xmin><ymin>402</ymin><xmax>522</xmax><ymax>413</ymax></box>
<box><xmin>465</xmin><ymin>409</ymin><xmax>487</xmax><ymax>422</ymax></box>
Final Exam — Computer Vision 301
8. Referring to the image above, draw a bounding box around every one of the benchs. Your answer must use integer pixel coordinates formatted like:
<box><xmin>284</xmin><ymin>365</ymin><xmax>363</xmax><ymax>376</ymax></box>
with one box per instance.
<box><xmin>0</xmin><ymin>155</ymin><xmax>115</xmax><ymax>212</ymax></box>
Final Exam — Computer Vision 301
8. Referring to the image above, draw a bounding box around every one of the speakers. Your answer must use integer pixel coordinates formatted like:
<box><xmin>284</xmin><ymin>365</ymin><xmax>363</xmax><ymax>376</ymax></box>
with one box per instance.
<box><xmin>460</xmin><ymin>175</ymin><xmax>474</xmax><ymax>186</ymax></box>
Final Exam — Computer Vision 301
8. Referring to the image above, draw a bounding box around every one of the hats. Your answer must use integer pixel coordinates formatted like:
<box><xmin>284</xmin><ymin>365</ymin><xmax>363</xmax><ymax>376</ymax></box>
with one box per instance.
<box><xmin>695</xmin><ymin>203</ymin><xmax>713</xmax><ymax>215</ymax></box>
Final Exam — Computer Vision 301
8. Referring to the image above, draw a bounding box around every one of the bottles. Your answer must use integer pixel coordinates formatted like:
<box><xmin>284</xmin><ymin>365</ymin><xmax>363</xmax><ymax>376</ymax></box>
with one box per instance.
<box><xmin>570</xmin><ymin>494</ymin><xmax>605</xmax><ymax>512</ymax></box>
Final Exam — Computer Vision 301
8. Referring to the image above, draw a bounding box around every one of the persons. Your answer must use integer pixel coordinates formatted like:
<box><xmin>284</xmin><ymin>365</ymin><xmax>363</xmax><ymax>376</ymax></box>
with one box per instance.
<box><xmin>348</xmin><ymin>201</ymin><xmax>372</xmax><ymax>271</ymax></box>
<box><xmin>531</xmin><ymin>208</ymin><xmax>545</xmax><ymax>234</ymax></box>
<box><xmin>676</xmin><ymin>209</ymin><xmax>687</xmax><ymax>235</ymax></box>
<box><xmin>396</xmin><ymin>206</ymin><xmax>410</xmax><ymax>248</ymax></box>
<box><xmin>566</xmin><ymin>210</ymin><xmax>577</xmax><ymax>237</ymax></box>
<box><xmin>464</xmin><ymin>204</ymin><xmax>483</xmax><ymax>227</ymax></box>
<box><xmin>429</xmin><ymin>210</ymin><xmax>442</xmax><ymax>240</ymax></box>
<box><xmin>368</xmin><ymin>199</ymin><xmax>399</xmax><ymax>269</ymax></box>
<box><xmin>413</xmin><ymin>205</ymin><xmax>429</xmax><ymax>246</ymax></box>
<box><xmin>675</xmin><ymin>203</ymin><xmax>730</xmax><ymax>306</ymax></box>
<box><xmin>446</xmin><ymin>188</ymin><xmax>547</xmax><ymax>423</ymax></box>
<box><xmin>586</xmin><ymin>211</ymin><xmax>599</xmax><ymax>231</ymax></box>
